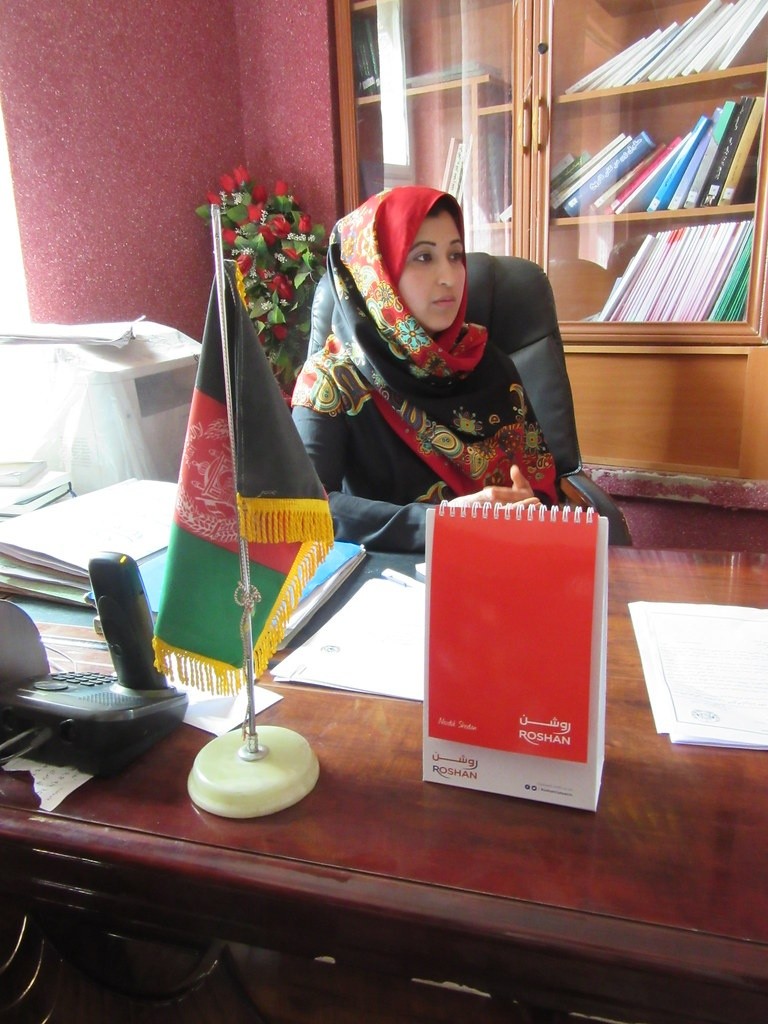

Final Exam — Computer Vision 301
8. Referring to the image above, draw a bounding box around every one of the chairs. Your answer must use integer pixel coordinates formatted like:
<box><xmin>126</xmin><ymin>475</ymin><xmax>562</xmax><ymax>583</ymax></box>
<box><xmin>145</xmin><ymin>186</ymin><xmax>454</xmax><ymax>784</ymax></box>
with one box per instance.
<box><xmin>306</xmin><ymin>251</ymin><xmax>635</xmax><ymax>547</ymax></box>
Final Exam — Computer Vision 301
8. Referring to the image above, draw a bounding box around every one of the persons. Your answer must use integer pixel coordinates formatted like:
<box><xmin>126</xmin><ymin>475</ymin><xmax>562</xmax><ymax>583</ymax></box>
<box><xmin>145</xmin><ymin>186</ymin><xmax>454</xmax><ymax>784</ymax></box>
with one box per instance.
<box><xmin>294</xmin><ymin>184</ymin><xmax>595</xmax><ymax>553</ymax></box>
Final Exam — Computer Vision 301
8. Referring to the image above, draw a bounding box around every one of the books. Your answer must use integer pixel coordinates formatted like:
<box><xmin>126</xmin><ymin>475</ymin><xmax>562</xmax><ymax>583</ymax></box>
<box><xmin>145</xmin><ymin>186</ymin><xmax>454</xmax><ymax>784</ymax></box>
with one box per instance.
<box><xmin>1</xmin><ymin>461</ymin><xmax>362</xmax><ymax>650</ymax></box>
<box><xmin>440</xmin><ymin>135</ymin><xmax>508</xmax><ymax>223</ymax></box>
<box><xmin>485</xmin><ymin>94</ymin><xmax>767</xmax><ymax>223</ymax></box>
<box><xmin>597</xmin><ymin>219</ymin><xmax>753</xmax><ymax>322</ymax></box>
<box><xmin>565</xmin><ymin>0</ymin><xmax>768</xmax><ymax>94</ymax></box>
<box><xmin>351</xmin><ymin>17</ymin><xmax>501</xmax><ymax>98</ymax></box>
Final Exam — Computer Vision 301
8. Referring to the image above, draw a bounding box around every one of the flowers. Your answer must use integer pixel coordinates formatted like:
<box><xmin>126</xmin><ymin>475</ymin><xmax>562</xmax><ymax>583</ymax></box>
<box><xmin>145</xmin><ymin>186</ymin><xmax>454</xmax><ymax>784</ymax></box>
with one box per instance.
<box><xmin>192</xmin><ymin>169</ymin><xmax>327</xmax><ymax>393</ymax></box>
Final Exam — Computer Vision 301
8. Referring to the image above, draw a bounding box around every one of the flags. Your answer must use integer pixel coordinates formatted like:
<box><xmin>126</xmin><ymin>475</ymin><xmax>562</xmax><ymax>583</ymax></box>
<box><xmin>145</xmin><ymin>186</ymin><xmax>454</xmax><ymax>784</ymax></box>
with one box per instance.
<box><xmin>152</xmin><ymin>261</ymin><xmax>334</xmax><ymax>697</ymax></box>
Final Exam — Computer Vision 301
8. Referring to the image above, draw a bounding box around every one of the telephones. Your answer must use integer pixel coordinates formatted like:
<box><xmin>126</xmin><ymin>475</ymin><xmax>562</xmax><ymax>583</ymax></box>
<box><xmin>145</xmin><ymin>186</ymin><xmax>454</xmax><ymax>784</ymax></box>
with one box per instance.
<box><xmin>0</xmin><ymin>551</ymin><xmax>189</xmax><ymax>769</ymax></box>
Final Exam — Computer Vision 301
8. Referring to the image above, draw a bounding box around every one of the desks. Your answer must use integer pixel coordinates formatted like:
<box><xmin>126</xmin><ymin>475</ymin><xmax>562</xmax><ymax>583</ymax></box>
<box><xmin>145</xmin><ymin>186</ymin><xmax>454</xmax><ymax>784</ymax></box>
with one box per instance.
<box><xmin>0</xmin><ymin>545</ymin><xmax>768</xmax><ymax>1024</ymax></box>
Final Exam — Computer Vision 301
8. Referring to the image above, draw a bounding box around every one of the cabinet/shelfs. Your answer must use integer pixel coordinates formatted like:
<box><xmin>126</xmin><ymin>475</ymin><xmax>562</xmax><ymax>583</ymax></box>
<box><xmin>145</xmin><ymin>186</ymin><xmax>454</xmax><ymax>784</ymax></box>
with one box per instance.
<box><xmin>333</xmin><ymin>0</ymin><xmax>768</xmax><ymax>510</ymax></box>
